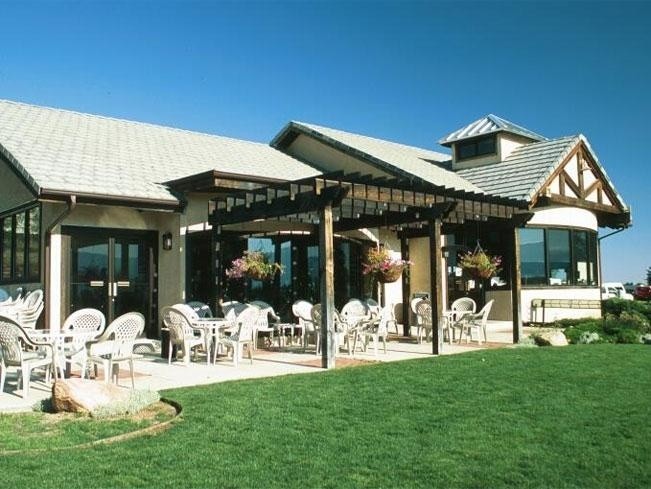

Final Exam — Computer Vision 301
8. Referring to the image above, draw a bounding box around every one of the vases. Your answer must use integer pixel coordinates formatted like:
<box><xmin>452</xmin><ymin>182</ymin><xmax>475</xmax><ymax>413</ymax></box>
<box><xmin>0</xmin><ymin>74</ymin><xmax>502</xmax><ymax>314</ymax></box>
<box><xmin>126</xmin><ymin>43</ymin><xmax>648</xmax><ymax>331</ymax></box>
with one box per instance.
<box><xmin>252</xmin><ymin>269</ymin><xmax>270</xmax><ymax>282</ymax></box>
<box><xmin>378</xmin><ymin>269</ymin><xmax>400</xmax><ymax>284</ymax></box>
<box><xmin>474</xmin><ymin>268</ymin><xmax>491</xmax><ymax>280</ymax></box>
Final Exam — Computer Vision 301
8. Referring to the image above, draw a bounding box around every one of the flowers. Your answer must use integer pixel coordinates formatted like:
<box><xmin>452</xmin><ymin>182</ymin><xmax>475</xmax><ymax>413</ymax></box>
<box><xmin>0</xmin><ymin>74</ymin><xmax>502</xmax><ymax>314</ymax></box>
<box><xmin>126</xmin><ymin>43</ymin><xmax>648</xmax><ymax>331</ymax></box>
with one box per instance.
<box><xmin>361</xmin><ymin>248</ymin><xmax>415</xmax><ymax>275</ymax></box>
<box><xmin>223</xmin><ymin>245</ymin><xmax>289</xmax><ymax>279</ymax></box>
<box><xmin>457</xmin><ymin>249</ymin><xmax>502</xmax><ymax>272</ymax></box>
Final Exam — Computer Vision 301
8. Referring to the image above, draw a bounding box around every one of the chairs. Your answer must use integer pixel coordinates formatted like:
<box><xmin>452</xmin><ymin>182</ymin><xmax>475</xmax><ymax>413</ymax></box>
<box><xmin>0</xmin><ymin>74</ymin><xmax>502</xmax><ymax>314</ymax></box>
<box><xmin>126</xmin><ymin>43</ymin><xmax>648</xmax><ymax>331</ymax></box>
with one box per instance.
<box><xmin>161</xmin><ymin>298</ymin><xmax>281</xmax><ymax>363</ymax></box>
<box><xmin>410</xmin><ymin>292</ymin><xmax>495</xmax><ymax>348</ymax></box>
<box><xmin>0</xmin><ymin>289</ymin><xmax>146</xmax><ymax>401</ymax></box>
<box><xmin>292</xmin><ymin>293</ymin><xmax>398</xmax><ymax>358</ymax></box>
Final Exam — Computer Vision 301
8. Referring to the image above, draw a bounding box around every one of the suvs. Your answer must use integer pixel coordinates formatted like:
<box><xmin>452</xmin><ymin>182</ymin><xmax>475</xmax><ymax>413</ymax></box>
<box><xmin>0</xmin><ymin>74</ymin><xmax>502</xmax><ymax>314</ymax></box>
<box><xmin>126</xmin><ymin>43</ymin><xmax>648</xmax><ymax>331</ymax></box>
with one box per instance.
<box><xmin>601</xmin><ymin>285</ymin><xmax>634</xmax><ymax>301</ymax></box>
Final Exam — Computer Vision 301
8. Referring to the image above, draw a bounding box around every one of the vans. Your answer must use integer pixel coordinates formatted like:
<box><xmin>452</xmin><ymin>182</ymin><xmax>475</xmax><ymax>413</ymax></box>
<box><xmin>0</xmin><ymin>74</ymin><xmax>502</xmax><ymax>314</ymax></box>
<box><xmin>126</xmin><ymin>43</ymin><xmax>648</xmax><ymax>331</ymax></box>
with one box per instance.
<box><xmin>602</xmin><ymin>282</ymin><xmax>627</xmax><ymax>294</ymax></box>
<box><xmin>633</xmin><ymin>284</ymin><xmax>651</xmax><ymax>299</ymax></box>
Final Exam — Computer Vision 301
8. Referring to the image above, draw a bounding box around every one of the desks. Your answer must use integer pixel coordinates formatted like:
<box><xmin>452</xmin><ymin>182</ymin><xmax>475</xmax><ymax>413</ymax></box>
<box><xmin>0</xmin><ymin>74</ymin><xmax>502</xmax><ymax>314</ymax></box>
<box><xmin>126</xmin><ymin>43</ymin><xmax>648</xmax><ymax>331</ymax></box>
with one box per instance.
<box><xmin>282</xmin><ymin>323</ymin><xmax>303</xmax><ymax>354</ymax></box>
